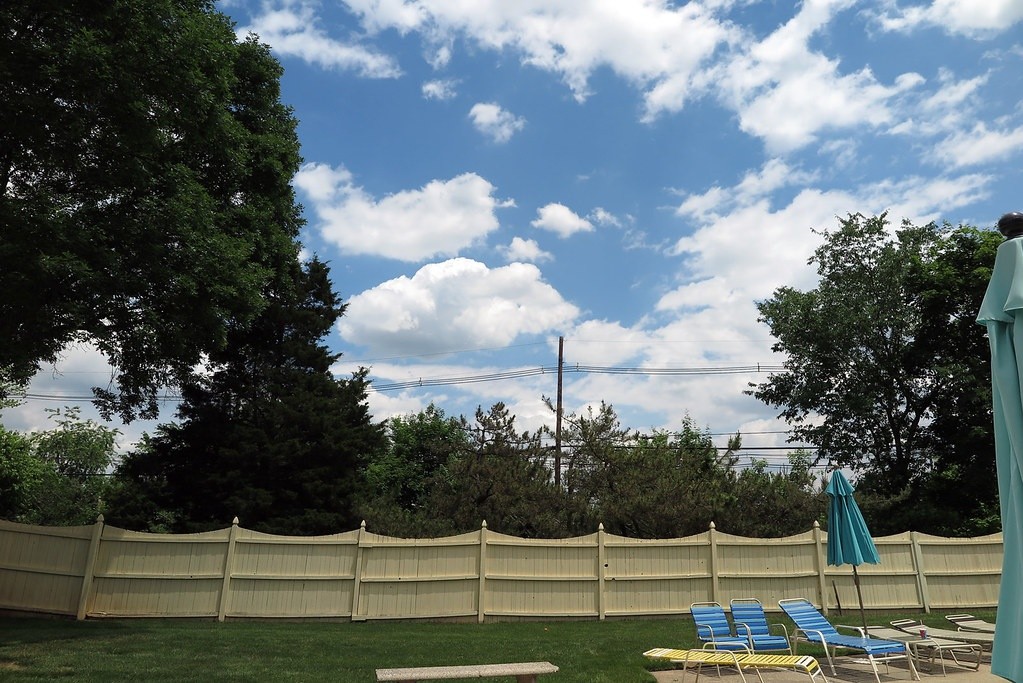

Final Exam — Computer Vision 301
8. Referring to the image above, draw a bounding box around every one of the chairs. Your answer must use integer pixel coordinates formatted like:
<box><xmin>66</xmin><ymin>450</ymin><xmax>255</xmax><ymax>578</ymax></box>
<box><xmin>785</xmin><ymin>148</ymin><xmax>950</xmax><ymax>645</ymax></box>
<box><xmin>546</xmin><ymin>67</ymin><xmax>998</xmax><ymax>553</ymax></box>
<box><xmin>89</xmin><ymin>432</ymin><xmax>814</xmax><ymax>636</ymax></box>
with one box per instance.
<box><xmin>946</xmin><ymin>612</ymin><xmax>997</xmax><ymax>652</ymax></box>
<box><xmin>691</xmin><ymin>601</ymin><xmax>755</xmax><ymax>677</ymax></box>
<box><xmin>730</xmin><ymin>599</ymin><xmax>797</xmax><ymax>671</ymax></box>
<box><xmin>854</xmin><ymin>622</ymin><xmax>982</xmax><ymax>675</ymax></box>
<box><xmin>779</xmin><ymin>598</ymin><xmax>914</xmax><ymax>683</ymax></box>
<box><xmin>644</xmin><ymin>647</ymin><xmax>830</xmax><ymax>683</ymax></box>
<box><xmin>889</xmin><ymin>619</ymin><xmax>996</xmax><ymax>666</ymax></box>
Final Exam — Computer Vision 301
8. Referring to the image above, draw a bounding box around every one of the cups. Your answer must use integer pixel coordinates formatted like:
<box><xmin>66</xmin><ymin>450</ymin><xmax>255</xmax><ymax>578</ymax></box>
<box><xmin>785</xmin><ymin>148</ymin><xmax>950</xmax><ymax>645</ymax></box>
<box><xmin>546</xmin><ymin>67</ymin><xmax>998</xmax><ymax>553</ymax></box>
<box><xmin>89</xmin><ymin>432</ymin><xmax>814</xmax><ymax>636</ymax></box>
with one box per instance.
<box><xmin>919</xmin><ymin>629</ymin><xmax>927</xmax><ymax>640</ymax></box>
